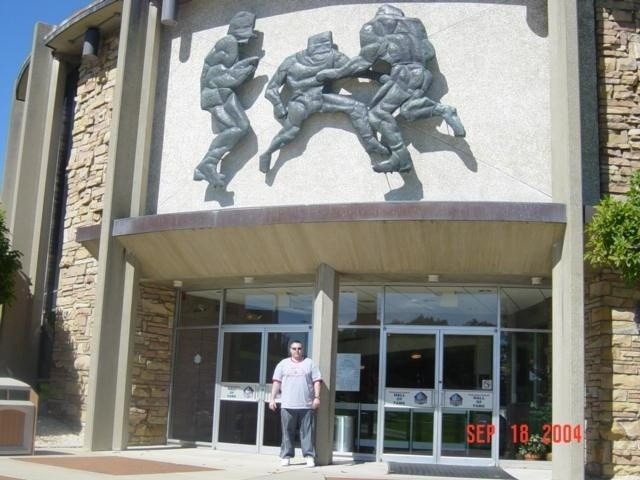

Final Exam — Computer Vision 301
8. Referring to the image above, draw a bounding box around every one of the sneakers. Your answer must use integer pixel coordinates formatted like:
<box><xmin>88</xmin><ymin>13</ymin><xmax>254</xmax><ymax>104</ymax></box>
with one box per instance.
<box><xmin>307</xmin><ymin>457</ymin><xmax>315</xmax><ymax>468</ymax></box>
<box><xmin>280</xmin><ymin>458</ymin><xmax>290</xmax><ymax>466</ymax></box>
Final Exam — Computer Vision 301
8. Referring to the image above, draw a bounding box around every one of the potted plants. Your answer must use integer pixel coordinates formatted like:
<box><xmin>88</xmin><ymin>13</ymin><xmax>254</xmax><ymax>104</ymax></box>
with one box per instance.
<box><xmin>517</xmin><ymin>433</ymin><xmax>550</xmax><ymax>460</ymax></box>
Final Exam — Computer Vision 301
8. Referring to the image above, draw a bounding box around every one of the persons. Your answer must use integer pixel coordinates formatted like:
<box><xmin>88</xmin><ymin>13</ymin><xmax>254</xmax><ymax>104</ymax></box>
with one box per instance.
<box><xmin>190</xmin><ymin>10</ymin><xmax>261</xmax><ymax>193</ymax></box>
<box><xmin>314</xmin><ymin>3</ymin><xmax>466</xmax><ymax>175</ymax></box>
<box><xmin>268</xmin><ymin>339</ymin><xmax>324</xmax><ymax>469</ymax></box>
<box><xmin>259</xmin><ymin>31</ymin><xmax>394</xmax><ymax>175</ymax></box>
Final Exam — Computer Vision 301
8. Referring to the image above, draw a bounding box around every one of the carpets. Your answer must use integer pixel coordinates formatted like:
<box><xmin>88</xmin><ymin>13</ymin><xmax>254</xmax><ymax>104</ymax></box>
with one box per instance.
<box><xmin>387</xmin><ymin>460</ymin><xmax>518</xmax><ymax>480</ymax></box>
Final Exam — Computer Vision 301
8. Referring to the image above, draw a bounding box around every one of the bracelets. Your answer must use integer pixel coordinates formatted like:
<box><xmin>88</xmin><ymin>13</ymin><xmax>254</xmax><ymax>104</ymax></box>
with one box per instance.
<box><xmin>314</xmin><ymin>396</ymin><xmax>321</xmax><ymax>399</ymax></box>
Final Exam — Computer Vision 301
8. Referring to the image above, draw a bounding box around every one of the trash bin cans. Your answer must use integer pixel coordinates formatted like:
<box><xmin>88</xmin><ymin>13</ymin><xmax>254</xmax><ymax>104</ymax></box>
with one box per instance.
<box><xmin>333</xmin><ymin>414</ymin><xmax>356</xmax><ymax>452</ymax></box>
<box><xmin>0</xmin><ymin>377</ymin><xmax>40</xmax><ymax>456</ymax></box>
